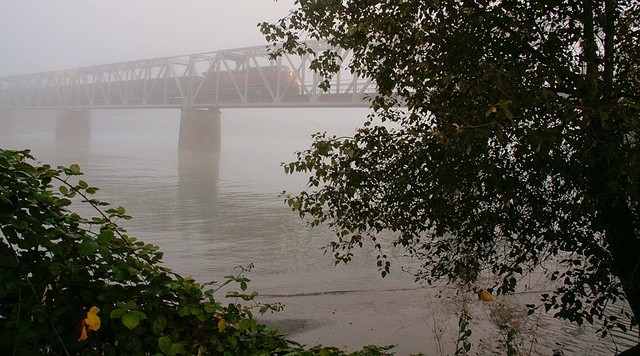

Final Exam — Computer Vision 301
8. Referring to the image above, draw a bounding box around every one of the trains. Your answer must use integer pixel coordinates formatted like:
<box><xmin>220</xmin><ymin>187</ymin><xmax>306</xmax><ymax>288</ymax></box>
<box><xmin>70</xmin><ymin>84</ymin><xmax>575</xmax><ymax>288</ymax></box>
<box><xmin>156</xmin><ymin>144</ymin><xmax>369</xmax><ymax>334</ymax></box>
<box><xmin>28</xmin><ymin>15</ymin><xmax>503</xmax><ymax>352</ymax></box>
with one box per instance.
<box><xmin>0</xmin><ymin>65</ymin><xmax>299</xmax><ymax>106</ymax></box>
